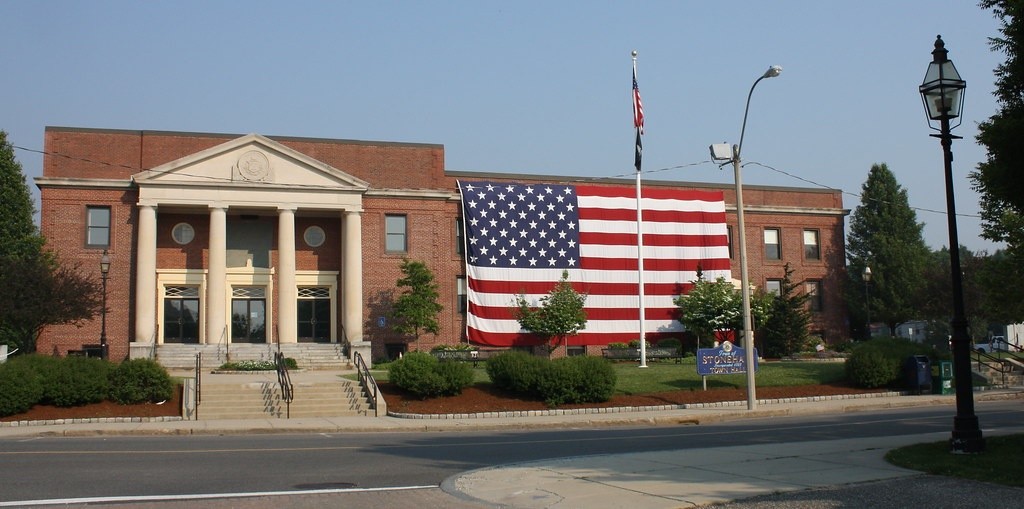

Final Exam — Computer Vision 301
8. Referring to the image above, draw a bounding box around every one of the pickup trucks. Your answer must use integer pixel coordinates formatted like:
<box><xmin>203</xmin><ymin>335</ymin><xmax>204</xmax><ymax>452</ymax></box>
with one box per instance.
<box><xmin>974</xmin><ymin>336</ymin><xmax>1005</xmax><ymax>353</ymax></box>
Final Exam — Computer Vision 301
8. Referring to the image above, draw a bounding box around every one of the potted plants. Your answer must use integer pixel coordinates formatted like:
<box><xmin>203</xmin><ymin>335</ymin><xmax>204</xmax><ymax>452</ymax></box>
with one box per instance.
<box><xmin>432</xmin><ymin>344</ymin><xmax>481</xmax><ymax>368</ymax></box>
<box><xmin>601</xmin><ymin>340</ymin><xmax>681</xmax><ymax>363</ymax></box>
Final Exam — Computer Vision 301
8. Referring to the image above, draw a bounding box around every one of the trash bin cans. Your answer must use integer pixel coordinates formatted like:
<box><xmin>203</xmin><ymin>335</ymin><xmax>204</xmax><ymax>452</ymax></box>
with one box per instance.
<box><xmin>384</xmin><ymin>341</ymin><xmax>408</xmax><ymax>363</ymax></box>
<box><xmin>909</xmin><ymin>355</ymin><xmax>932</xmax><ymax>395</ymax></box>
<box><xmin>82</xmin><ymin>344</ymin><xmax>110</xmax><ymax>360</ymax></box>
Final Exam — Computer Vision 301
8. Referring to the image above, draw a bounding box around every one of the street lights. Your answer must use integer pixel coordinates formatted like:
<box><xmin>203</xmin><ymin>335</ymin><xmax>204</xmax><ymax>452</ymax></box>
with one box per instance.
<box><xmin>98</xmin><ymin>249</ymin><xmax>112</xmax><ymax>361</ymax></box>
<box><xmin>733</xmin><ymin>65</ymin><xmax>783</xmax><ymax>408</ymax></box>
<box><xmin>862</xmin><ymin>265</ymin><xmax>872</xmax><ymax>340</ymax></box>
<box><xmin>918</xmin><ymin>35</ymin><xmax>986</xmax><ymax>454</ymax></box>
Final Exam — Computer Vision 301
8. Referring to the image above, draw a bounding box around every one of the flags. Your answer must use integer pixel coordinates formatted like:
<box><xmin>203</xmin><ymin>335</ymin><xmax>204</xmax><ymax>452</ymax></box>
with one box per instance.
<box><xmin>457</xmin><ymin>180</ymin><xmax>735</xmax><ymax>346</ymax></box>
<box><xmin>631</xmin><ymin>70</ymin><xmax>644</xmax><ymax>171</ymax></box>
